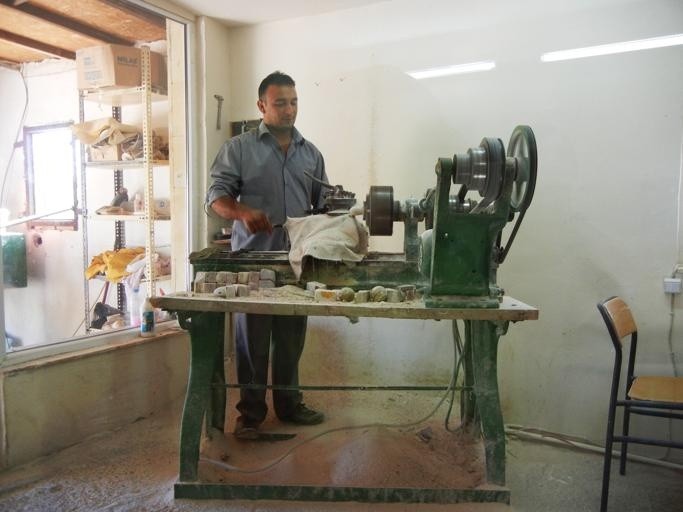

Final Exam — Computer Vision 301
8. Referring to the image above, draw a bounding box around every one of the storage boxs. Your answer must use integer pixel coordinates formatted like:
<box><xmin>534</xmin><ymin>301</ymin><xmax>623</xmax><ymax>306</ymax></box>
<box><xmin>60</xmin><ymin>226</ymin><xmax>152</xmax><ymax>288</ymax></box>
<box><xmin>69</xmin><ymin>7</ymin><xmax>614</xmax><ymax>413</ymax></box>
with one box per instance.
<box><xmin>75</xmin><ymin>43</ymin><xmax>164</xmax><ymax>90</ymax></box>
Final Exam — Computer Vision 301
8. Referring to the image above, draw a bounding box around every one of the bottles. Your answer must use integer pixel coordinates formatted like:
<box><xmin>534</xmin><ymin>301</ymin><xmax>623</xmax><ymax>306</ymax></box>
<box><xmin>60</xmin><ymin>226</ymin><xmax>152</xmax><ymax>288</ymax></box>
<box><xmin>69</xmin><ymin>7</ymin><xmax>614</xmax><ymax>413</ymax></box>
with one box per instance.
<box><xmin>140</xmin><ymin>294</ymin><xmax>156</xmax><ymax>338</ymax></box>
<box><xmin>131</xmin><ymin>286</ymin><xmax>143</xmax><ymax>327</ymax></box>
<box><xmin>134</xmin><ymin>194</ymin><xmax>144</xmax><ymax>213</ymax></box>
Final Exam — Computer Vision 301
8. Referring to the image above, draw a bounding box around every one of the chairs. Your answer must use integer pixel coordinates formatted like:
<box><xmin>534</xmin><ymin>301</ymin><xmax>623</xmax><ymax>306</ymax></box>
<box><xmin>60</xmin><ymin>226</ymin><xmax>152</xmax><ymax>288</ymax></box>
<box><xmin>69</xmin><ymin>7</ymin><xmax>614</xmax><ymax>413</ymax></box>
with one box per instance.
<box><xmin>598</xmin><ymin>295</ymin><xmax>683</xmax><ymax>511</ymax></box>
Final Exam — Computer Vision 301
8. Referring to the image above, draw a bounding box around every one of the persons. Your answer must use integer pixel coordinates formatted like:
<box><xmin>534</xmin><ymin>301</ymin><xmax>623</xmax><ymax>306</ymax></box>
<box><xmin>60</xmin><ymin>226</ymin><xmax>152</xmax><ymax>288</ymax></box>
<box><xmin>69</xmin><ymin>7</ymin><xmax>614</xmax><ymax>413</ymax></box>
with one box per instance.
<box><xmin>204</xmin><ymin>69</ymin><xmax>331</xmax><ymax>444</ymax></box>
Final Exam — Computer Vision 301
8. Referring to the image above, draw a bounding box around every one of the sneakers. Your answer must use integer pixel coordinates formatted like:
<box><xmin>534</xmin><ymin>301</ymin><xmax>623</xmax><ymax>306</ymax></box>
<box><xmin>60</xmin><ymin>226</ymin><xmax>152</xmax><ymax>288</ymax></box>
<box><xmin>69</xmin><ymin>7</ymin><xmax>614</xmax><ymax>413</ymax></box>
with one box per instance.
<box><xmin>280</xmin><ymin>403</ymin><xmax>323</xmax><ymax>425</ymax></box>
<box><xmin>235</xmin><ymin>416</ymin><xmax>258</xmax><ymax>440</ymax></box>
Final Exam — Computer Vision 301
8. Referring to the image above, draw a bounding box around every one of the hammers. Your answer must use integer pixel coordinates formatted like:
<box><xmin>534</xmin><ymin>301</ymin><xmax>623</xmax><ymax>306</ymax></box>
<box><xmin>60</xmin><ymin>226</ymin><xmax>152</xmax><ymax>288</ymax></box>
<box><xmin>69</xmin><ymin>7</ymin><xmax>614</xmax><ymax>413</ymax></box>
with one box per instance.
<box><xmin>214</xmin><ymin>95</ymin><xmax>224</xmax><ymax>129</ymax></box>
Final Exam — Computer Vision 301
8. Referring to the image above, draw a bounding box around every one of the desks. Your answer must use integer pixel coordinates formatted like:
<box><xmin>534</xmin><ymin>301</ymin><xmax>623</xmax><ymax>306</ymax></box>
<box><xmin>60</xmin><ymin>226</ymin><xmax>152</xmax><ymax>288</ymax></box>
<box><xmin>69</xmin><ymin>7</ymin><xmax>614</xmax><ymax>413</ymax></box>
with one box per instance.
<box><xmin>151</xmin><ymin>292</ymin><xmax>539</xmax><ymax>503</ymax></box>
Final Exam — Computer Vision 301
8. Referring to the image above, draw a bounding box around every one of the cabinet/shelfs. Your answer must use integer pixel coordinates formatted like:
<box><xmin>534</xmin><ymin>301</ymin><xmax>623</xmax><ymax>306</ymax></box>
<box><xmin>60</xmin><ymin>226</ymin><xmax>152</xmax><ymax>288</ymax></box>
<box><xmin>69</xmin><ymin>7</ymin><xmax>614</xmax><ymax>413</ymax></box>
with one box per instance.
<box><xmin>77</xmin><ymin>42</ymin><xmax>174</xmax><ymax>335</ymax></box>
